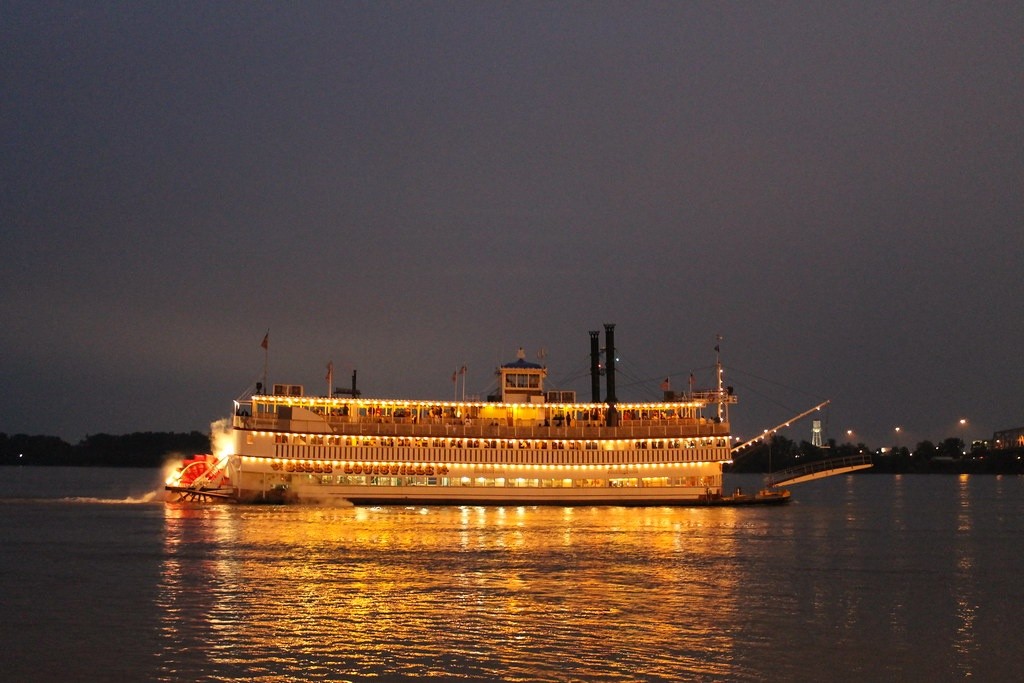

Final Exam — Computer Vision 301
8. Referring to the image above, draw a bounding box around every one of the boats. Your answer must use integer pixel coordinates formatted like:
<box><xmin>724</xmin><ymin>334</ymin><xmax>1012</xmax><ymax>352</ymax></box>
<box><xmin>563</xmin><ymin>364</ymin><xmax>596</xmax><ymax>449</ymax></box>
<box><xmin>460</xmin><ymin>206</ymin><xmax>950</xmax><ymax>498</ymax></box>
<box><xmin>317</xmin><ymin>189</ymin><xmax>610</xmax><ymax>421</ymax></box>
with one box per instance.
<box><xmin>162</xmin><ymin>323</ymin><xmax>874</xmax><ymax>506</ymax></box>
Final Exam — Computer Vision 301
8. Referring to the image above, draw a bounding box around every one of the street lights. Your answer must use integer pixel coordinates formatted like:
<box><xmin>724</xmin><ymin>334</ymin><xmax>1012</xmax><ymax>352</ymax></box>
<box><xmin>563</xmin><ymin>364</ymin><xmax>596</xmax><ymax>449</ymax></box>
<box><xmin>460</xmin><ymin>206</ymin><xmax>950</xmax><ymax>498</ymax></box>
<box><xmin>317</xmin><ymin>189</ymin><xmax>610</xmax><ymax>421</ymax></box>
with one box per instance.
<box><xmin>894</xmin><ymin>427</ymin><xmax>900</xmax><ymax>448</ymax></box>
<box><xmin>959</xmin><ymin>419</ymin><xmax>966</xmax><ymax>448</ymax></box>
<box><xmin>847</xmin><ymin>430</ymin><xmax>852</xmax><ymax>445</ymax></box>
